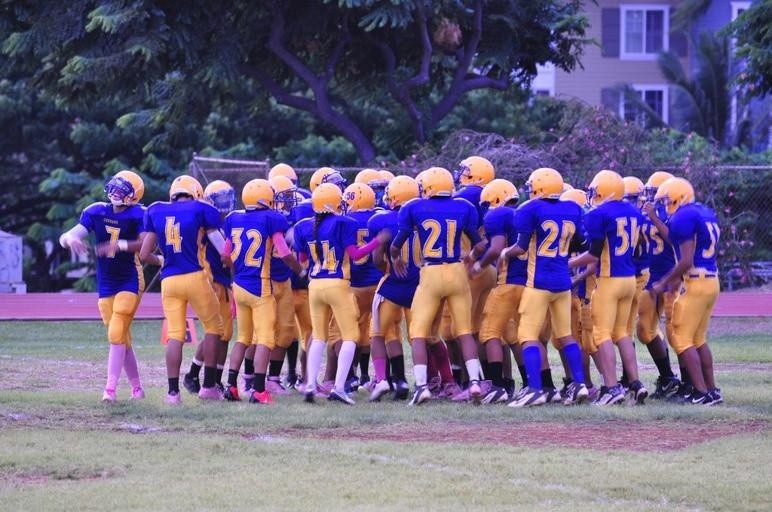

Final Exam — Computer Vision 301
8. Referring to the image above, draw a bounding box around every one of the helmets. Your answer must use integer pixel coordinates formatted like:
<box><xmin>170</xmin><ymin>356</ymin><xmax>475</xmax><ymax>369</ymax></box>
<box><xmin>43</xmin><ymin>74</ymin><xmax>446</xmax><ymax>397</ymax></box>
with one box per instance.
<box><xmin>312</xmin><ymin>183</ymin><xmax>342</xmax><ymax>214</ymax></box>
<box><xmin>453</xmin><ymin>156</ymin><xmax>495</xmax><ymax>187</ymax></box>
<box><xmin>655</xmin><ymin>177</ymin><xmax>694</xmax><ymax>214</ymax></box>
<box><xmin>104</xmin><ymin>170</ymin><xmax>145</xmax><ymax>206</ymax></box>
<box><xmin>310</xmin><ymin>168</ymin><xmax>347</xmax><ymax>192</ymax></box>
<box><xmin>204</xmin><ymin>180</ymin><xmax>236</xmax><ymax>213</ymax></box>
<box><xmin>242</xmin><ymin>178</ymin><xmax>275</xmax><ymax>210</ymax></box>
<box><xmin>376</xmin><ymin>170</ymin><xmax>393</xmax><ymax>185</ymax></box>
<box><xmin>355</xmin><ymin>170</ymin><xmax>376</xmax><ymax>183</ymax></box>
<box><xmin>525</xmin><ymin>168</ymin><xmax>563</xmax><ymax>200</ymax></box>
<box><xmin>343</xmin><ymin>182</ymin><xmax>376</xmax><ymax>211</ymax></box>
<box><xmin>623</xmin><ymin>176</ymin><xmax>644</xmax><ymax>199</ymax></box>
<box><xmin>589</xmin><ymin>170</ymin><xmax>625</xmax><ymax>206</ymax></box>
<box><xmin>169</xmin><ymin>175</ymin><xmax>203</xmax><ymax>201</ymax></box>
<box><xmin>560</xmin><ymin>189</ymin><xmax>587</xmax><ymax>207</ymax></box>
<box><xmin>644</xmin><ymin>171</ymin><xmax>674</xmax><ymax>202</ymax></box>
<box><xmin>416</xmin><ymin>167</ymin><xmax>454</xmax><ymax>200</ymax></box>
<box><xmin>479</xmin><ymin>179</ymin><xmax>518</xmax><ymax>208</ymax></box>
<box><xmin>385</xmin><ymin>176</ymin><xmax>419</xmax><ymax>207</ymax></box>
<box><xmin>271</xmin><ymin>176</ymin><xmax>298</xmax><ymax>209</ymax></box>
<box><xmin>268</xmin><ymin>163</ymin><xmax>298</xmax><ymax>188</ymax></box>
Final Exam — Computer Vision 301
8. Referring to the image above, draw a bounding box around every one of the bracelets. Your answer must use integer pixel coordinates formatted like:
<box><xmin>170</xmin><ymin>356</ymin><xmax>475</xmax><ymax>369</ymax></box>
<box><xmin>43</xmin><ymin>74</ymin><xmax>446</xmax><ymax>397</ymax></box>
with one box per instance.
<box><xmin>116</xmin><ymin>239</ymin><xmax>129</xmax><ymax>253</ymax></box>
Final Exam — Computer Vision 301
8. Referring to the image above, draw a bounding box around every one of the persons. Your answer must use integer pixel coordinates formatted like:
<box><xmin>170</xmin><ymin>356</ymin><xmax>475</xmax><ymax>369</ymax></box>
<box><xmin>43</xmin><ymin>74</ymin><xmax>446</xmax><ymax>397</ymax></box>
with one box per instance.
<box><xmin>140</xmin><ymin>157</ymin><xmax>724</xmax><ymax>409</ymax></box>
<box><xmin>60</xmin><ymin>170</ymin><xmax>159</xmax><ymax>400</ymax></box>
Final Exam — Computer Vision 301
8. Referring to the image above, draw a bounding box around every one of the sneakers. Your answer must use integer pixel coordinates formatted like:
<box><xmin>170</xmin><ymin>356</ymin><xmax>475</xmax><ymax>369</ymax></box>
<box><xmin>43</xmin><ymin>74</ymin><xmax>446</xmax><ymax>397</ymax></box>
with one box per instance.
<box><xmin>543</xmin><ymin>387</ymin><xmax>561</xmax><ymax>403</ymax></box>
<box><xmin>470</xmin><ymin>380</ymin><xmax>481</xmax><ymax>401</ymax></box>
<box><xmin>183</xmin><ymin>373</ymin><xmax>199</xmax><ymax>394</ymax></box>
<box><xmin>434</xmin><ymin>383</ymin><xmax>459</xmax><ymax>398</ymax></box>
<box><xmin>316</xmin><ymin>381</ymin><xmax>334</xmax><ymax>394</ymax></box>
<box><xmin>563</xmin><ymin>382</ymin><xmax>588</xmax><ymax>405</ymax></box>
<box><xmin>408</xmin><ymin>385</ymin><xmax>431</xmax><ymax>405</ymax></box>
<box><xmin>686</xmin><ymin>390</ymin><xmax>715</xmax><ymax>406</ymax></box>
<box><xmin>131</xmin><ymin>387</ymin><xmax>144</xmax><ymax>400</ymax></box>
<box><xmin>223</xmin><ymin>385</ymin><xmax>241</xmax><ymax>400</ymax></box>
<box><xmin>649</xmin><ymin>378</ymin><xmax>681</xmax><ymax>399</ymax></box>
<box><xmin>588</xmin><ymin>385</ymin><xmax>598</xmax><ymax>400</ymax></box>
<box><xmin>669</xmin><ymin>384</ymin><xmax>694</xmax><ymax>401</ymax></box>
<box><xmin>591</xmin><ymin>386</ymin><xmax>625</xmax><ymax>405</ymax></box>
<box><xmin>628</xmin><ymin>380</ymin><xmax>648</xmax><ymax>406</ymax></box>
<box><xmin>267</xmin><ymin>381</ymin><xmax>288</xmax><ymax>395</ymax></box>
<box><xmin>370</xmin><ymin>379</ymin><xmax>390</xmax><ymax>400</ymax></box>
<box><xmin>102</xmin><ymin>388</ymin><xmax>115</xmax><ymax>401</ymax></box>
<box><xmin>249</xmin><ymin>391</ymin><xmax>273</xmax><ymax>404</ymax></box>
<box><xmin>707</xmin><ymin>388</ymin><xmax>723</xmax><ymax>402</ymax></box>
<box><xmin>329</xmin><ymin>389</ymin><xmax>355</xmax><ymax>405</ymax></box>
<box><xmin>394</xmin><ymin>381</ymin><xmax>408</xmax><ymax>399</ymax></box>
<box><xmin>429</xmin><ymin>377</ymin><xmax>440</xmax><ymax>392</ymax></box>
<box><xmin>507</xmin><ymin>385</ymin><xmax>546</xmax><ymax>407</ymax></box>
<box><xmin>304</xmin><ymin>386</ymin><xmax>314</xmax><ymax>402</ymax></box>
<box><xmin>199</xmin><ymin>385</ymin><xmax>224</xmax><ymax>400</ymax></box>
<box><xmin>358</xmin><ymin>381</ymin><xmax>376</xmax><ymax>392</ymax></box>
<box><xmin>481</xmin><ymin>388</ymin><xmax>508</xmax><ymax>404</ymax></box>
<box><xmin>166</xmin><ymin>389</ymin><xmax>179</xmax><ymax>404</ymax></box>
<box><xmin>451</xmin><ymin>388</ymin><xmax>470</xmax><ymax>401</ymax></box>
<box><xmin>294</xmin><ymin>379</ymin><xmax>303</xmax><ymax>393</ymax></box>
